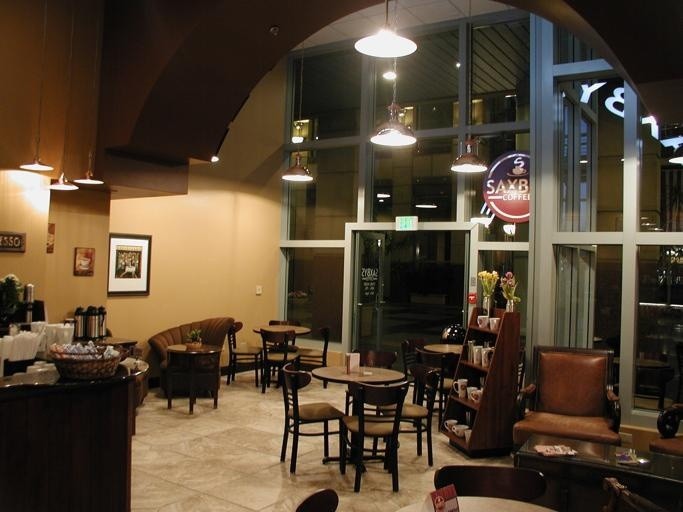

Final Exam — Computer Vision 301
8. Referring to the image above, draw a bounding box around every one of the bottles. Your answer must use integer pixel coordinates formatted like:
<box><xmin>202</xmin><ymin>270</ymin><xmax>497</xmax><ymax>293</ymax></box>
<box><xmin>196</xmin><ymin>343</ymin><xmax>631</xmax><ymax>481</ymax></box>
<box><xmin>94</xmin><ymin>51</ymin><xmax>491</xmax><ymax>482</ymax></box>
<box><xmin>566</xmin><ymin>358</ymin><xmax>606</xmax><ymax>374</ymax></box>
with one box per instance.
<box><xmin>466</xmin><ymin>340</ymin><xmax>475</xmax><ymax>363</ymax></box>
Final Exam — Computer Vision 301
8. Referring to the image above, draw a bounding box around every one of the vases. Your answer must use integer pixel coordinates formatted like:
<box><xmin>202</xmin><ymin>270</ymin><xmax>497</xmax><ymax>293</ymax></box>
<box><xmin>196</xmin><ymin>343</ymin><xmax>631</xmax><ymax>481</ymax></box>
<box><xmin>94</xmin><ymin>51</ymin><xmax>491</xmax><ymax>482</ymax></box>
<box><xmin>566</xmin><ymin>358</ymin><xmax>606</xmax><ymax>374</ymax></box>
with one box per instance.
<box><xmin>482</xmin><ymin>297</ymin><xmax>493</xmax><ymax>315</ymax></box>
<box><xmin>507</xmin><ymin>300</ymin><xmax>514</xmax><ymax>312</ymax></box>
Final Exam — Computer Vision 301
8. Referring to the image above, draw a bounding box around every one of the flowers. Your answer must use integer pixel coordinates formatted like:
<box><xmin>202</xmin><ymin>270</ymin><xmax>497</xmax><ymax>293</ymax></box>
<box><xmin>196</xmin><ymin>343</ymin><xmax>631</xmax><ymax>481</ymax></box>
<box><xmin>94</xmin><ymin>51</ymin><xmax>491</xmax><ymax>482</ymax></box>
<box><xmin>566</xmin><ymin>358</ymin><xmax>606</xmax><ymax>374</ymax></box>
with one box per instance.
<box><xmin>499</xmin><ymin>271</ymin><xmax>521</xmax><ymax>303</ymax></box>
<box><xmin>478</xmin><ymin>269</ymin><xmax>499</xmax><ymax>298</ymax></box>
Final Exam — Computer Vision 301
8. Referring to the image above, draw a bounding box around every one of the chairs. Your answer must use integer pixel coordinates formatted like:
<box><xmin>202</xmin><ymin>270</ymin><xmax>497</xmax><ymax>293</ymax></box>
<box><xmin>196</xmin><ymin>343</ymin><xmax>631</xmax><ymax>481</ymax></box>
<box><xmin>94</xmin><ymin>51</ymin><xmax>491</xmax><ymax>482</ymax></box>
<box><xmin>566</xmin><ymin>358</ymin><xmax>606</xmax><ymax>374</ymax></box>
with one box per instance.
<box><xmin>400</xmin><ymin>338</ymin><xmax>463</xmax><ymax>432</ymax></box>
<box><xmin>513</xmin><ymin>343</ymin><xmax>621</xmax><ymax>446</ymax></box>
<box><xmin>597</xmin><ymin>477</ymin><xmax>666</xmax><ymax>511</ymax></box>
<box><xmin>278</xmin><ymin>358</ymin><xmax>437</xmax><ymax>492</ymax></box>
<box><xmin>434</xmin><ymin>465</ymin><xmax>547</xmax><ymax>503</ymax></box>
<box><xmin>146</xmin><ymin>317</ymin><xmax>234</xmax><ymax>397</ymax></box>
<box><xmin>226</xmin><ymin>319</ymin><xmax>330</xmax><ymax>394</ymax></box>
<box><xmin>295</xmin><ymin>489</ymin><xmax>339</xmax><ymax>512</ymax></box>
<box><xmin>649</xmin><ymin>401</ymin><xmax>682</xmax><ymax>456</ymax></box>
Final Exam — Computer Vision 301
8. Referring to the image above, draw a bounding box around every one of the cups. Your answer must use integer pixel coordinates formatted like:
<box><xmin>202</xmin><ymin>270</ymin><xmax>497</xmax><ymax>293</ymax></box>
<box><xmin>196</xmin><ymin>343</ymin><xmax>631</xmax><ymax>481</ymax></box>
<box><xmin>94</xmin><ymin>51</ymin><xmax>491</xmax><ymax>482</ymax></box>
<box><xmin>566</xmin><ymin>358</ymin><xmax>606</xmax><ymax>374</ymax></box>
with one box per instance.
<box><xmin>451</xmin><ymin>425</ymin><xmax>469</xmax><ymax>438</ymax></box>
<box><xmin>463</xmin><ymin>430</ymin><xmax>471</xmax><ymax>443</ymax></box>
<box><xmin>488</xmin><ymin>317</ymin><xmax>500</xmax><ymax>331</ymax></box>
<box><xmin>13</xmin><ymin>360</ymin><xmax>56</xmax><ymax>376</ymax></box>
<box><xmin>443</xmin><ymin>419</ymin><xmax>458</xmax><ymax>433</ymax></box>
<box><xmin>472</xmin><ymin>345</ymin><xmax>481</xmax><ymax>365</ymax></box>
<box><xmin>452</xmin><ymin>378</ymin><xmax>468</xmax><ymax>398</ymax></box>
<box><xmin>480</xmin><ymin>347</ymin><xmax>490</xmax><ymax>368</ymax></box>
<box><xmin>476</xmin><ymin>316</ymin><xmax>488</xmax><ymax>329</ymax></box>
<box><xmin>466</xmin><ymin>386</ymin><xmax>482</xmax><ymax>405</ymax></box>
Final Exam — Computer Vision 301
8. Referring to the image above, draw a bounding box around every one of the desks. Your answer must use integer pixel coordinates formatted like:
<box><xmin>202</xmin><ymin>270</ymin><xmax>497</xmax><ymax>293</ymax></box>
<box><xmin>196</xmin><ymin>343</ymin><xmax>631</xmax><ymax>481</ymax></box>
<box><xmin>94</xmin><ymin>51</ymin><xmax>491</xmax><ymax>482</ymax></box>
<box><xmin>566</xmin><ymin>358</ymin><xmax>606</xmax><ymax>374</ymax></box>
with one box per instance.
<box><xmin>167</xmin><ymin>344</ymin><xmax>221</xmax><ymax>414</ymax></box>
<box><xmin>394</xmin><ymin>495</ymin><xmax>560</xmax><ymax>512</ymax></box>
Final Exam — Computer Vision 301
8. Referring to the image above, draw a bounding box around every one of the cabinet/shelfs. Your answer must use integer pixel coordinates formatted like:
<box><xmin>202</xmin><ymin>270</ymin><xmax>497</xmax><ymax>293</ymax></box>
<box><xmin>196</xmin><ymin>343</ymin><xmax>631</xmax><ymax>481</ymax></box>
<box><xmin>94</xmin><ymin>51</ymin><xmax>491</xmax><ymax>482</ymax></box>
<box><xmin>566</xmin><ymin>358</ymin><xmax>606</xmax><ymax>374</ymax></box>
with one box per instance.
<box><xmin>0</xmin><ymin>0</ymin><xmax>104</xmax><ymax>181</ymax></box>
<box><xmin>438</xmin><ymin>305</ymin><xmax>521</xmax><ymax>459</ymax></box>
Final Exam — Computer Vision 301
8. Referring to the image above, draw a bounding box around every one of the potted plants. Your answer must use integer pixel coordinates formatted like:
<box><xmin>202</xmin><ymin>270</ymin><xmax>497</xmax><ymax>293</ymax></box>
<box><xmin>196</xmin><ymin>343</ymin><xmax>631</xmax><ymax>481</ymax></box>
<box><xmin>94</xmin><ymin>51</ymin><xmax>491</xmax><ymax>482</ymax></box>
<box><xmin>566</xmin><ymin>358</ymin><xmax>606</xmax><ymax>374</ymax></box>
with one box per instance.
<box><xmin>188</xmin><ymin>328</ymin><xmax>202</xmax><ymax>350</ymax></box>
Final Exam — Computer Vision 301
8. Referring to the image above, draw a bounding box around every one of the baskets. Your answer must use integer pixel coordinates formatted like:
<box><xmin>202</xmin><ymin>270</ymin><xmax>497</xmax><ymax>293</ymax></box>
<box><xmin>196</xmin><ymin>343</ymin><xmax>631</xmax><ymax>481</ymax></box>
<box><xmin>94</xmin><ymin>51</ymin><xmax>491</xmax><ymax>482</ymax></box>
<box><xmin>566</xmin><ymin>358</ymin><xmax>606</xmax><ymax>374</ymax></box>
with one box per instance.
<box><xmin>52</xmin><ymin>346</ymin><xmax>130</xmax><ymax>380</ymax></box>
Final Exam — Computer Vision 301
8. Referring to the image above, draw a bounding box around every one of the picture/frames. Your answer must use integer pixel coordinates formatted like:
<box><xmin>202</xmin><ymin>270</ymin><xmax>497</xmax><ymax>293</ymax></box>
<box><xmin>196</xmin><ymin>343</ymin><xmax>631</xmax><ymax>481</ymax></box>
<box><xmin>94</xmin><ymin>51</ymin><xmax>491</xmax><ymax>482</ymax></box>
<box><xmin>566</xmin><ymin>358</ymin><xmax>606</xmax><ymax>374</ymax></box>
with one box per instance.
<box><xmin>106</xmin><ymin>233</ymin><xmax>152</xmax><ymax>297</ymax></box>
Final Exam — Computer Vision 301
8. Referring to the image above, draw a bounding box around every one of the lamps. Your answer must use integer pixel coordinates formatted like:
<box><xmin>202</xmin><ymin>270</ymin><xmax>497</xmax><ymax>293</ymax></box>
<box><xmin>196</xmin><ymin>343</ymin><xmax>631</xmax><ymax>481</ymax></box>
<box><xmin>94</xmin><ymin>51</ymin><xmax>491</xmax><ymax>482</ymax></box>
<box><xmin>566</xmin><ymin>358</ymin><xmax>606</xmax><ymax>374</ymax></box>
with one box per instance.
<box><xmin>282</xmin><ymin>39</ymin><xmax>313</xmax><ymax>182</ymax></box>
<box><xmin>354</xmin><ymin>0</ymin><xmax>418</xmax><ymax>58</ymax></box>
<box><xmin>18</xmin><ymin>0</ymin><xmax>104</xmax><ymax>192</ymax></box>
<box><xmin>451</xmin><ymin>2</ymin><xmax>488</xmax><ymax>173</ymax></box>
<box><xmin>370</xmin><ymin>58</ymin><xmax>418</xmax><ymax>147</ymax></box>
